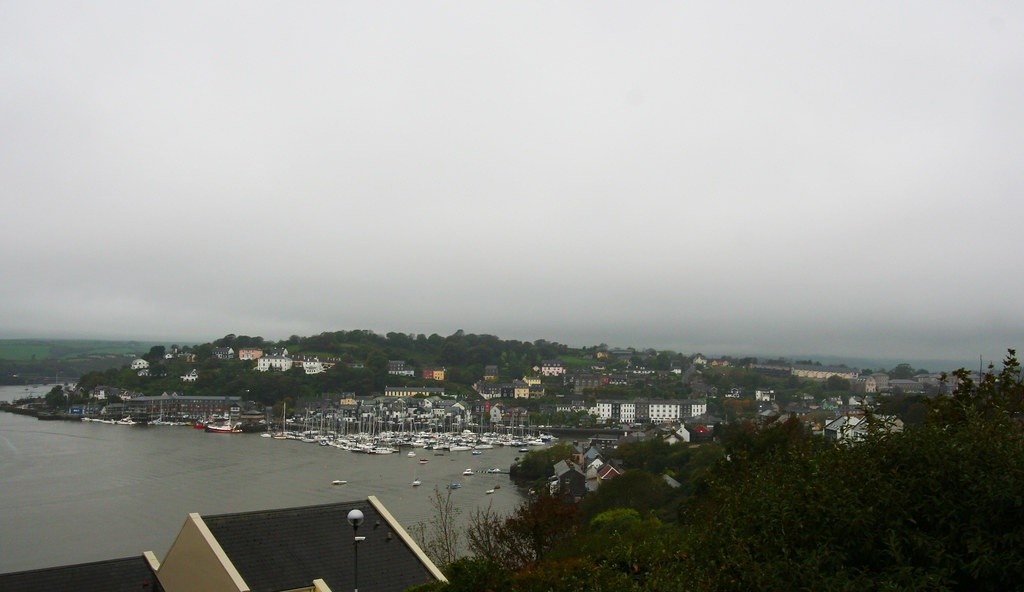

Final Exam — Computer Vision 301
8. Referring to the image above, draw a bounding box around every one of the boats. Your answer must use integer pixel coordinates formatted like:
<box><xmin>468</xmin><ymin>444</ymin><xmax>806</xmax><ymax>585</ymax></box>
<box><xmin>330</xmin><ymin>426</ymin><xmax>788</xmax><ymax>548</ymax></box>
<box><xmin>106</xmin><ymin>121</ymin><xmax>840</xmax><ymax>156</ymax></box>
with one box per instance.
<box><xmin>80</xmin><ymin>396</ymin><xmax>560</xmax><ymax>501</ymax></box>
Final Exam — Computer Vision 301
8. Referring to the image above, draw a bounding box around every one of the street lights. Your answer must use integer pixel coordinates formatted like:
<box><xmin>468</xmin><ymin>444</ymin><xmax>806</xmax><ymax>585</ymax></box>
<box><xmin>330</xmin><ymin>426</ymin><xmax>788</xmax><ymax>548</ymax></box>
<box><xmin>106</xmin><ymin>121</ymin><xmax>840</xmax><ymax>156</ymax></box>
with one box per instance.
<box><xmin>347</xmin><ymin>508</ymin><xmax>364</xmax><ymax>592</ymax></box>
